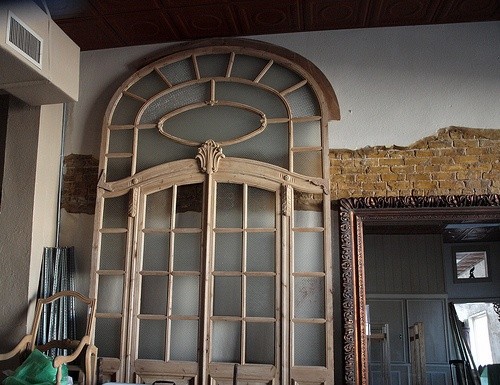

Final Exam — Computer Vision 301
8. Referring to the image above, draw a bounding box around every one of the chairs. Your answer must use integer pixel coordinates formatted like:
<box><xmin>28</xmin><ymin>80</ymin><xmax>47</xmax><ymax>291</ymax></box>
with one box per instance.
<box><xmin>0</xmin><ymin>290</ymin><xmax>99</xmax><ymax>385</ymax></box>
